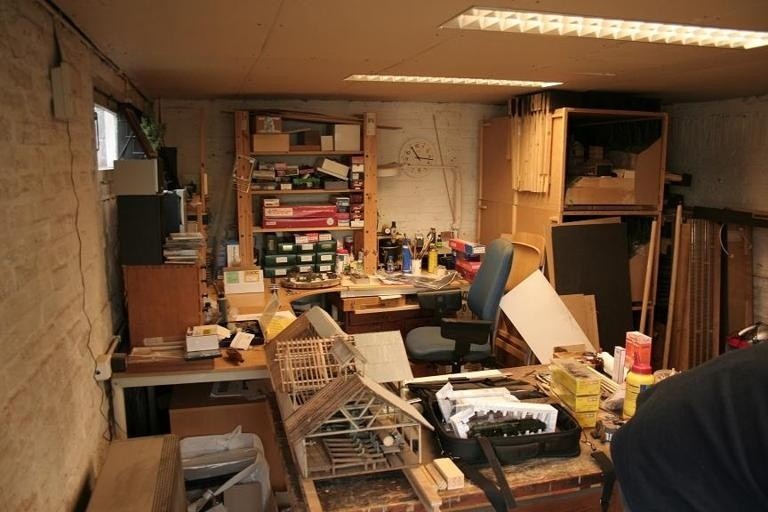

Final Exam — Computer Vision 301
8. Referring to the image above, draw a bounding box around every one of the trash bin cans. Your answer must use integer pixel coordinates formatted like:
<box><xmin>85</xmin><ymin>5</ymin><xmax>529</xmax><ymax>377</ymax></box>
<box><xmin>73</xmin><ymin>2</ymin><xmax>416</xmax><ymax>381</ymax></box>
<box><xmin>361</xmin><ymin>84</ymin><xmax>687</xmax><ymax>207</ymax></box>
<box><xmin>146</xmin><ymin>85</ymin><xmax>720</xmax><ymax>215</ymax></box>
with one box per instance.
<box><xmin>179</xmin><ymin>433</ymin><xmax>280</xmax><ymax>512</ymax></box>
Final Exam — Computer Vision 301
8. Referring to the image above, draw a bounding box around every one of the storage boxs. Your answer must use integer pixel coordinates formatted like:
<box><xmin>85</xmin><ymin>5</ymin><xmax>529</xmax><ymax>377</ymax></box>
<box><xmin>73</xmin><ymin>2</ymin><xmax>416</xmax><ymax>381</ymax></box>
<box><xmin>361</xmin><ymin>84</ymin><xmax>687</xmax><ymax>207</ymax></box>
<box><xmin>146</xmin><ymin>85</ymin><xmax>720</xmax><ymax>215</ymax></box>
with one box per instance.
<box><xmin>251</xmin><ymin>115</ymin><xmax>364</xmax><ymax>278</ymax></box>
<box><xmin>164</xmin><ymin>388</ymin><xmax>287</xmax><ymax>490</ymax></box>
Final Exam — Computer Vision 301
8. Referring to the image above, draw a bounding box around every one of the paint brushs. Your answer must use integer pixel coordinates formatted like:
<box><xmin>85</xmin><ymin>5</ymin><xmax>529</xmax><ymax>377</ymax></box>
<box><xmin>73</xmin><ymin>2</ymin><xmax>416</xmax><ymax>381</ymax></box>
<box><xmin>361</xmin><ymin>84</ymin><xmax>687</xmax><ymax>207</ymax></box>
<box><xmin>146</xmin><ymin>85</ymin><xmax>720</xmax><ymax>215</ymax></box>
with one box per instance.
<box><xmin>404</xmin><ymin>233</ymin><xmax>433</xmax><ymax>260</ymax></box>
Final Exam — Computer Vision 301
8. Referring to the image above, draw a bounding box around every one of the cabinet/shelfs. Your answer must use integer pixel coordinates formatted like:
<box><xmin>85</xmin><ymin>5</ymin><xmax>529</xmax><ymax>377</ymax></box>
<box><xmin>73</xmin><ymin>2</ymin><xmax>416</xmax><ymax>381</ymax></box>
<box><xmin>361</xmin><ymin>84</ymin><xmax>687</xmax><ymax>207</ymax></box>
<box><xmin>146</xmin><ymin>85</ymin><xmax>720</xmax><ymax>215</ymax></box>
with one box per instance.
<box><xmin>231</xmin><ymin>108</ymin><xmax>378</xmax><ymax>278</ymax></box>
<box><xmin>82</xmin><ymin>433</ymin><xmax>186</xmax><ymax>512</ymax></box>
<box><xmin>474</xmin><ymin>112</ymin><xmax>516</xmax><ymax>244</ymax></box>
<box><xmin>516</xmin><ymin>106</ymin><xmax>671</xmax><ymax>363</ymax></box>
<box><xmin>121</xmin><ymin>253</ymin><xmax>206</xmax><ymax>350</ymax></box>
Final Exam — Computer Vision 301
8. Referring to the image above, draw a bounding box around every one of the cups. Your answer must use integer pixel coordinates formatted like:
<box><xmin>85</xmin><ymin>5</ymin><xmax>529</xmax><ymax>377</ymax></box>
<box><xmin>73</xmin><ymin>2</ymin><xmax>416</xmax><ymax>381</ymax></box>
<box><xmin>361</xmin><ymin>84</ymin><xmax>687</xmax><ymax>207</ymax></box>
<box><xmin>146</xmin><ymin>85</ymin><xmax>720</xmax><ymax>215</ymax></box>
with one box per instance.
<box><xmin>411</xmin><ymin>260</ymin><xmax>422</xmax><ymax>274</ymax></box>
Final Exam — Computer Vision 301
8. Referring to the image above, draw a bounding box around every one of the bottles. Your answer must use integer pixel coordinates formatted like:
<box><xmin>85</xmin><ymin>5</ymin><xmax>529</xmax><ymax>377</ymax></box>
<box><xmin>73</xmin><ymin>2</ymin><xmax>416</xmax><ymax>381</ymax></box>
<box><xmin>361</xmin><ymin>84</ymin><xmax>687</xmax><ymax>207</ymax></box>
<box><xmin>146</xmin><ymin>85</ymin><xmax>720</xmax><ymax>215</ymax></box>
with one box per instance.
<box><xmin>344</xmin><ymin>236</ymin><xmax>354</xmax><ymax>257</ymax></box>
<box><xmin>429</xmin><ymin>228</ymin><xmax>437</xmax><ymax>249</ymax></box>
<box><xmin>621</xmin><ymin>365</ymin><xmax>655</xmax><ymax>420</ymax></box>
<box><xmin>402</xmin><ymin>246</ymin><xmax>411</xmax><ymax>272</ymax></box>
<box><xmin>386</xmin><ymin>256</ymin><xmax>395</xmax><ymax>273</ymax></box>
<box><xmin>390</xmin><ymin>221</ymin><xmax>397</xmax><ymax>244</ymax></box>
<box><xmin>336</xmin><ymin>250</ymin><xmax>349</xmax><ymax>274</ymax></box>
<box><xmin>436</xmin><ymin>235</ymin><xmax>442</xmax><ymax>248</ymax></box>
<box><xmin>428</xmin><ymin>245</ymin><xmax>437</xmax><ymax>273</ymax></box>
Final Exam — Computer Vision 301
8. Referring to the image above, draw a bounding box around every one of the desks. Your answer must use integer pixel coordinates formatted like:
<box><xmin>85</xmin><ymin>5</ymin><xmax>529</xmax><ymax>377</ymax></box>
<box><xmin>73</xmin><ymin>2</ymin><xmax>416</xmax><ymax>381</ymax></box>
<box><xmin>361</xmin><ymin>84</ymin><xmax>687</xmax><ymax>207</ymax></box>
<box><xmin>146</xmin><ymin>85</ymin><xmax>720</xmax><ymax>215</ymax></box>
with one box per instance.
<box><xmin>108</xmin><ymin>342</ymin><xmax>273</xmax><ymax>443</ymax></box>
<box><xmin>279</xmin><ymin>360</ymin><xmax>624</xmax><ymax>512</ymax></box>
<box><xmin>213</xmin><ymin>271</ymin><xmax>470</xmax><ymax>364</ymax></box>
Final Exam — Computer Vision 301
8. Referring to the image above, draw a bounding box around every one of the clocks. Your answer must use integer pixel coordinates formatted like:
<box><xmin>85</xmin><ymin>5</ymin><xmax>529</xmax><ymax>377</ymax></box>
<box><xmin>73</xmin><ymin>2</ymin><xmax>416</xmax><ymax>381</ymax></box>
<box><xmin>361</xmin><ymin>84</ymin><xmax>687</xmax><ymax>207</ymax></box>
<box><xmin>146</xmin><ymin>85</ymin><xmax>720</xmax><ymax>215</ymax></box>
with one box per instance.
<box><xmin>398</xmin><ymin>136</ymin><xmax>438</xmax><ymax>179</ymax></box>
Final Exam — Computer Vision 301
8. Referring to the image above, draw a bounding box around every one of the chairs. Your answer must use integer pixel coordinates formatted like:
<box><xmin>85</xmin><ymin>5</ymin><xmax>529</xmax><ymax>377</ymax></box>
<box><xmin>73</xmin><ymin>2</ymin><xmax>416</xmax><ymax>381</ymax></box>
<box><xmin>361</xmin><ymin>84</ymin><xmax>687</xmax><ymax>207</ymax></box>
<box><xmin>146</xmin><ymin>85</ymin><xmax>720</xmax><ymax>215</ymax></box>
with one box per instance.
<box><xmin>401</xmin><ymin>237</ymin><xmax>514</xmax><ymax>374</ymax></box>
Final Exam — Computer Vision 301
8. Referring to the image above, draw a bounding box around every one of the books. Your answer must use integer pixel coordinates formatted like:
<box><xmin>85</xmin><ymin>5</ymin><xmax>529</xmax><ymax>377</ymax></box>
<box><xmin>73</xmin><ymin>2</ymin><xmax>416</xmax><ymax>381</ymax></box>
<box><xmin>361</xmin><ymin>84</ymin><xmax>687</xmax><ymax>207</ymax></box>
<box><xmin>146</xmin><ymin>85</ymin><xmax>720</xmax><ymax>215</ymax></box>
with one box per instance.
<box><xmin>163</xmin><ymin>232</ymin><xmax>205</xmax><ymax>265</ymax></box>
